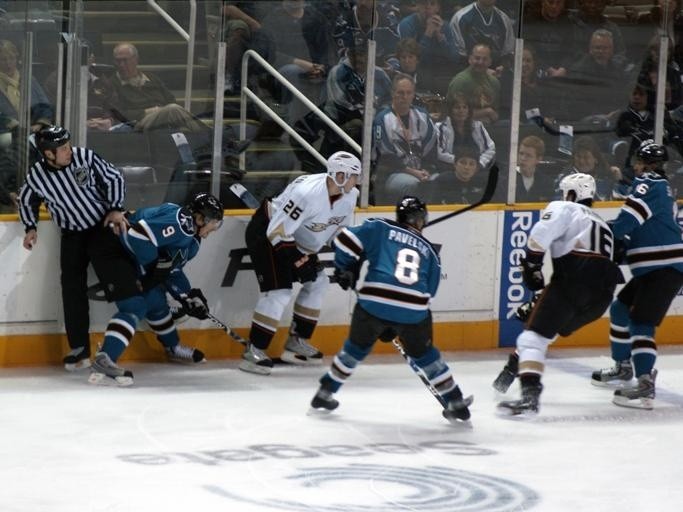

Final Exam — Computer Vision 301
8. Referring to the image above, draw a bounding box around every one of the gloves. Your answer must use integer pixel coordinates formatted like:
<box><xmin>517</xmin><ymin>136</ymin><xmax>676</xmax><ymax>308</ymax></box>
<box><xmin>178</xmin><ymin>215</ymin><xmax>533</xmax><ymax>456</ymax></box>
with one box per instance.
<box><xmin>335</xmin><ymin>270</ymin><xmax>355</xmax><ymax>289</ymax></box>
<box><xmin>519</xmin><ymin>258</ymin><xmax>543</xmax><ymax>290</ymax></box>
<box><xmin>183</xmin><ymin>297</ymin><xmax>209</xmax><ymax>319</ymax></box>
<box><xmin>295</xmin><ymin>256</ymin><xmax>318</xmax><ymax>283</ymax></box>
<box><xmin>515</xmin><ymin>294</ymin><xmax>542</xmax><ymax>320</ymax></box>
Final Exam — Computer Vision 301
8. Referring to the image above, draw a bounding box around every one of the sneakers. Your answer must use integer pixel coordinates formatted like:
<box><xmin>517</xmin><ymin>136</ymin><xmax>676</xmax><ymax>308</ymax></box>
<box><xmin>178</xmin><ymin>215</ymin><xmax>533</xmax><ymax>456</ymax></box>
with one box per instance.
<box><xmin>64</xmin><ymin>346</ymin><xmax>92</xmax><ymax>363</ymax></box>
<box><xmin>166</xmin><ymin>345</ymin><xmax>207</xmax><ymax>362</ymax></box>
<box><xmin>280</xmin><ymin>335</ymin><xmax>323</xmax><ymax>358</ymax></box>
<box><xmin>238</xmin><ymin>344</ymin><xmax>272</xmax><ymax>366</ymax></box>
<box><xmin>443</xmin><ymin>405</ymin><xmax>470</xmax><ymax>420</ymax></box>
<box><xmin>591</xmin><ymin>361</ymin><xmax>633</xmax><ymax>382</ymax></box>
<box><xmin>306</xmin><ymin>391</ymin><xmax>338</xmax><ymax>409</ymax></box>
<box><xmin>88</xmin><ymin>351</ymin><xmax>134</xmax><ymax>378</ymax></box>
<box><xmin>494</xmin><ymin>355</ymin><xmax>517</xmax><ymax>393</ymax></box>
<box><xmin>613</xmin><ymin>370</ymin><xmax>657</xmax><ymax>399</ymax></box>
<box><xmin>497</xmin><ymin>394</ymin><xmax>538</xmax><ymax>415</ymax></box>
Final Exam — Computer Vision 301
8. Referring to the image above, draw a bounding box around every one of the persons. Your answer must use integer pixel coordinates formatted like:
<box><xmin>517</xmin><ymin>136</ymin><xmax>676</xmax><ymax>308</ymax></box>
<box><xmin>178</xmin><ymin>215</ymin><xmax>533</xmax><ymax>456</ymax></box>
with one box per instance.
<box><xmin>238</xmin><ymin>150</ymin><xmax>363</xmax><ymax>375</ymax></box>
<box><xmin>173</xmin><ymin>1</ymin><xmax>682</xmax><ymax>207</ymax></box>
<box><xmin>311</xmin><ymin>197</ymin><xmax>474</xmax><ymax>420</ymax></box>
<box><xmin>493</xmin><ymin>172</ymin><xmax>624</xmax><ymax>415</ymax></box>
<box><xmin>87</xmin><ymin>192</ymin><xmax>224</xmax><ymax>385</ymax></box>
<box><xmin>603</xmin><ymin>140</ymin><xmax>683</xmax><ymax>410</ymax></box>
<box><xmin>19</xmin><ymin>123</ymin><xmax>191</xmax><ymax>373</ymax></box>
<box><xmin>0</xmin><ymin>38</ymin><xmax>250</xmax><ymax>205</ymax></box>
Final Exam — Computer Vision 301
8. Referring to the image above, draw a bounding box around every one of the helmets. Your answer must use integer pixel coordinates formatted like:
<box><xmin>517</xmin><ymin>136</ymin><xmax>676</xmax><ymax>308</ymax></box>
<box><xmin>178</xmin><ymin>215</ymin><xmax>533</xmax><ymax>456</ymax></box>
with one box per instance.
<box><xmin>36</xmin><ymin>125</ymin><xmax>70</xmax><ymax>151</ymax></box>
<box><xmin>558</xmin><ymin>172</ymin><xmax>596</xmax><ymax>202</ymax></box>
<box><xmin>397</xmin><ymin>197</ymin><xmax>426</xmax><ymax>223</ymax></box>
<box><xmin>192</xmin><ymin>193</ymin><xmax>223</xmax><ymax>221</ymax></box>
<box><xmin>326</xmin><ymin>151</ymin><xmax>361</xmax><ymax>186</ymax></box>
<box><xmin>636</xmin><ymin>143</ymin><xmax>668</xmax><ymax>164</ymax></box>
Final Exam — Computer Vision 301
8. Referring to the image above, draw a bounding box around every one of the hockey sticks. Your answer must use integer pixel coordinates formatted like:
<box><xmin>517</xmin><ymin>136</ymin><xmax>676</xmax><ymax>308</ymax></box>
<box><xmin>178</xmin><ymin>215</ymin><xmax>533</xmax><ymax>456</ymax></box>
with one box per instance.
<box><xmin>423</xmin><ymin>164</ymin><xmax>499</xmax><ymax>228</ymax></box>
<box><xmin>165</xmin><ymin>279</ymin><xmax>250</xmax><ymax>350</ymax></box>
<box><xmin>352</xmin><ymin>286</ymin><xmax>474</xmax><ymax>419</ymax></box>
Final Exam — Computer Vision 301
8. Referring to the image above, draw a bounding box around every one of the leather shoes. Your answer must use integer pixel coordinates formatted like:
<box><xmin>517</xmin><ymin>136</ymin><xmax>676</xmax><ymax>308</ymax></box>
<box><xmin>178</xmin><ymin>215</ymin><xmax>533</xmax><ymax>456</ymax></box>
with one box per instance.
<box><xmin>222</xmin><ymin>140</ymin><xmax>250</xmax><ymax>154</ymax></box>
